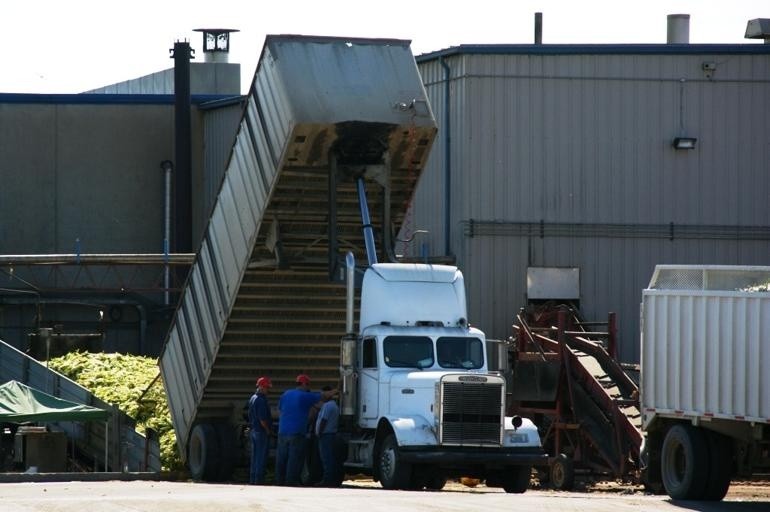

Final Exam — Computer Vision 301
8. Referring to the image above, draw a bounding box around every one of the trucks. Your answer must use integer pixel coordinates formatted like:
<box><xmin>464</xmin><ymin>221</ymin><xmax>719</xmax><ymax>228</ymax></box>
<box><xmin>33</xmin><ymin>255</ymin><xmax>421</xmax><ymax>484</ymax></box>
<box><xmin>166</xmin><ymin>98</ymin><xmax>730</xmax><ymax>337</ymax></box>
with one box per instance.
<box><xmin>157</xmin><ymin>33</ymin><xmax>549</xmax><ymax>494</ymax></box>
<box><xmin>638</xmin><ymin>263</ymin><xmax>770</xmax><ymax>500</ymax></box>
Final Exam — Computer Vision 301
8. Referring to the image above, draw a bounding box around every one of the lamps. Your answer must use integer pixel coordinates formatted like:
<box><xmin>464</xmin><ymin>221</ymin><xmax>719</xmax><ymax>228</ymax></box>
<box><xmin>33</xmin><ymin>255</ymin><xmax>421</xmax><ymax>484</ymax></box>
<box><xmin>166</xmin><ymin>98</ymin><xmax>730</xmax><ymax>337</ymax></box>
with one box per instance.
<box><xmin>674</xmin><ymin>137</ymin><xmax>696</xmax><ymax>149</ymax></box>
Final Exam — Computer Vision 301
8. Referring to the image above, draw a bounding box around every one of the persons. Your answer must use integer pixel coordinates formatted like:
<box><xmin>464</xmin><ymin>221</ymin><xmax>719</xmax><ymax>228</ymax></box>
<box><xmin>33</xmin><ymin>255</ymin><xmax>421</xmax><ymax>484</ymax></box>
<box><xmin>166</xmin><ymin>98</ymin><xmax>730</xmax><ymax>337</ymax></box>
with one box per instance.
<box><xmin>271</xmin><ymin>374</ymin><xmax>340</xmax><ymax>485</ymax></box>
<box><xmin>314</xmin><ymin>384</ymin><xmax>341</xmax><ymax>488</ymax></box>
<box><xmin>302</xmin><ymin>388</ymin><xmax>325</xmax><ymax>485</ymax></box>
<box><xmin>246</xmin><ymin>376</ymin><xmax>274</xmax><ymax>484</ymax></box>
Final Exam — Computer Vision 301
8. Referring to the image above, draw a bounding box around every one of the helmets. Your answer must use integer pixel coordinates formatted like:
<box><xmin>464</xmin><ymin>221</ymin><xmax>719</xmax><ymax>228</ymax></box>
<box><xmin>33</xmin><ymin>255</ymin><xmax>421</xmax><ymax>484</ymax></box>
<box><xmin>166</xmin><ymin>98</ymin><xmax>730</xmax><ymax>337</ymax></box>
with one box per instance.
<box><xmin>296</xmin><ymin>374</ymin><xmax>310</xmax><ymax>385</ymax></box>
<box><xmin>256</xmin><ymin>377</ymin><xmax>272</xmax><ymax>389</ymax></box>
